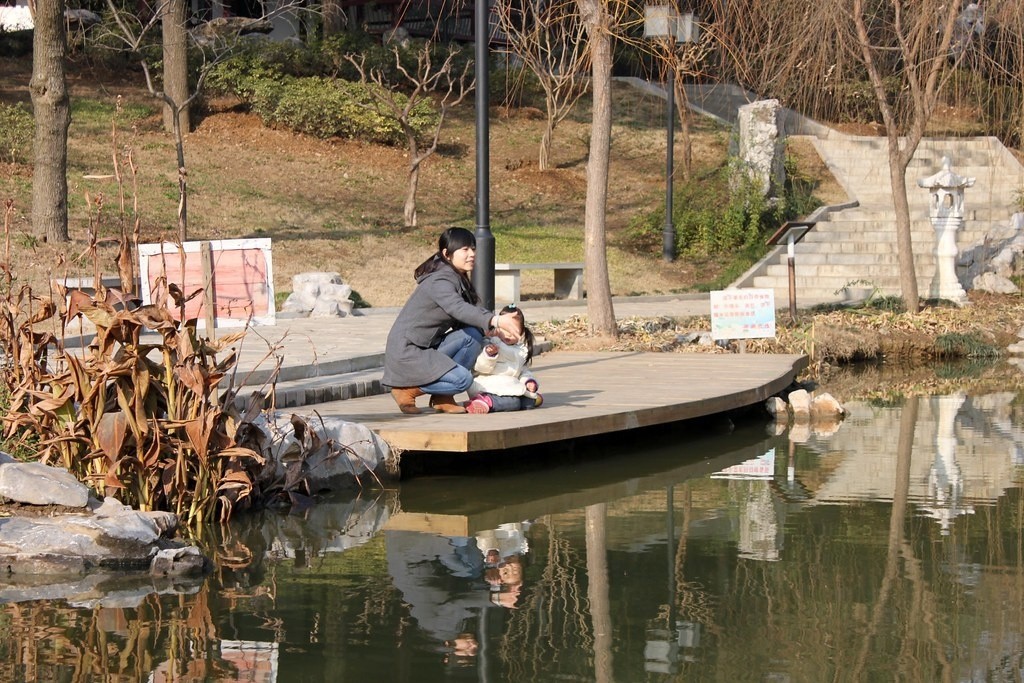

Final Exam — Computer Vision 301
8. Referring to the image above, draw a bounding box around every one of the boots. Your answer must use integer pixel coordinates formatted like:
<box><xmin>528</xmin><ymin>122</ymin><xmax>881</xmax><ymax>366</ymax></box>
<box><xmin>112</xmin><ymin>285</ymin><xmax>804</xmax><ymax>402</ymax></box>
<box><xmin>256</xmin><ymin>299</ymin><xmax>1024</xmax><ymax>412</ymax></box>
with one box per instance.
<box><xmin>430</xmin><ymin>394</ymin><xmax>466</xmax><ymax>413</ymax></box>
<box><xmin>390</xmin><ymin>386</ymin><xmax>425</xmax><ymax>413</ymax></box>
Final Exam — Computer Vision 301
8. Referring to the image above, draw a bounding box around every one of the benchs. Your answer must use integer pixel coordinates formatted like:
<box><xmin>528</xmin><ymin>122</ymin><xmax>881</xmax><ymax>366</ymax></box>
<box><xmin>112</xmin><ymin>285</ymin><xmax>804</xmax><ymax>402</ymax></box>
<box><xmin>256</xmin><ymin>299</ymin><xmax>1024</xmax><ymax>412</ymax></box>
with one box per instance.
<box><xmin>50</xmin><ymin>276</ymin><xmax>141</xmax><ymax>315</ymax></box>
<box><xmin>494</xmin><ymin>263</ymin><xmax>586</xmax><ymax>304</ymax></box>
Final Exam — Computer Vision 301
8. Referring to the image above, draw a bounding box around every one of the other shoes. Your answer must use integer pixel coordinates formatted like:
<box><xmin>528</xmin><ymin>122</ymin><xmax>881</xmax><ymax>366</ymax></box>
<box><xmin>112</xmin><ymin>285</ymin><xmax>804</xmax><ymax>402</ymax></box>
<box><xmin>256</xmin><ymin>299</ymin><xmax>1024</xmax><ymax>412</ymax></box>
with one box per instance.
<box><xmin>535</xmin><ymin>393</ymin><xmax>543</xmax><ymax>407</ymax></box>
<box><xmin>463</xmin><ymin>394</ymin><xmax>492</xmax><ymax>414</ymax></box>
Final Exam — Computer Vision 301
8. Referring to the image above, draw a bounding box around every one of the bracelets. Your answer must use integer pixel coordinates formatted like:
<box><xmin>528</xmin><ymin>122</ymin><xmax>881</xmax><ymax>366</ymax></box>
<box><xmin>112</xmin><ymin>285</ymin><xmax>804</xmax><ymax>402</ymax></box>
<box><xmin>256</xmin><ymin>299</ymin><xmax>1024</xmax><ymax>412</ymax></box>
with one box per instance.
<box><xmin>493</xmin><ymin>316</ymin><xmax>499</xmax><ymax>328</ymax></box>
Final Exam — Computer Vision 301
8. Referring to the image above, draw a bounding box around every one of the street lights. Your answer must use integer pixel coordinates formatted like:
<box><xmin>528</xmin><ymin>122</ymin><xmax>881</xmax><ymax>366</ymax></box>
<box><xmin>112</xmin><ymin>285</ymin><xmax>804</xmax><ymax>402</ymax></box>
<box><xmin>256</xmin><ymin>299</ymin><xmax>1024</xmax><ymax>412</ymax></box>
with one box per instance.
<box><xmin>643</xmin><ymin>5</ymin><xmax>700</xmax><ymax>263</ymax></box>
<box><xmin>643</xmin><ymin>486</ymin><xmax>701</xmax><ymax>674</ymax></box>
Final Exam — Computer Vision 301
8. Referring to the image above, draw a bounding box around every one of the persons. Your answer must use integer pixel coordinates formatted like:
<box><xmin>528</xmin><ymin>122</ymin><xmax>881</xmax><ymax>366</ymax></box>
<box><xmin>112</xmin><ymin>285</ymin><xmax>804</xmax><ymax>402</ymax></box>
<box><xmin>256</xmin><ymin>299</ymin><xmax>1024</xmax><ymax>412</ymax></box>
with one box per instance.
<box><xmin>463</xmin><ymin>303</ymin><xmax>543</xmax><ymax>414</ymax></box>
<box><xmin>384</xmin><ymin>519</ymin><xmax>532</xmax><ymax>670</ymax></box>
<box><xmin>381</xmin><ymin>227</ymin><xmax>521</xmax><ymax>414</ymax></box>
